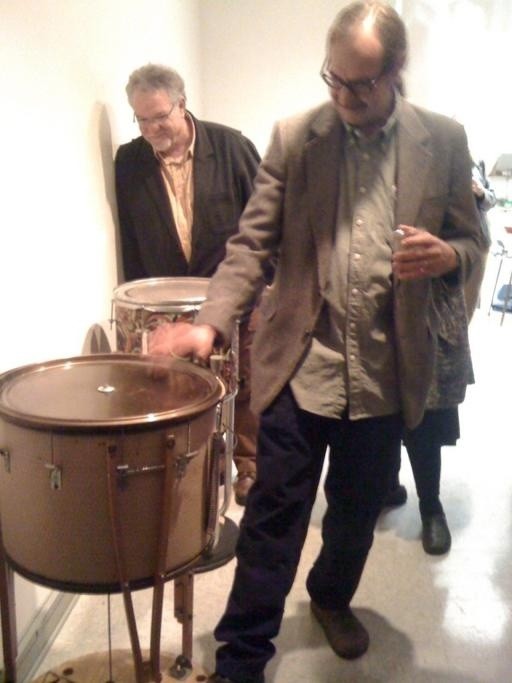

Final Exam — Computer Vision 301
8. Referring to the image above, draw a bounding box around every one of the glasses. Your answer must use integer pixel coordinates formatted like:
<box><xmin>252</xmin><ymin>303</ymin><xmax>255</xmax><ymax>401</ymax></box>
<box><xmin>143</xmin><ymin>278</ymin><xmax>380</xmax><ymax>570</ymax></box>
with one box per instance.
<box><xmin>317</xmin><ymin>54</ymin><xmax>394</xmax><ymax>91</ymax></box>
<box><xmin>125</xmin><ymin>102</ymin><xmax>182</xmax><ymax>126</ymax></box>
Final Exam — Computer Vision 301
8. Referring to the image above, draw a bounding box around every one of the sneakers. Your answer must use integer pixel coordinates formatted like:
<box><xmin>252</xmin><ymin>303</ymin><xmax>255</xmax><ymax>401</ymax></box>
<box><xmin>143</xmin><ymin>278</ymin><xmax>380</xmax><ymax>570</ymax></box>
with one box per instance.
<box><xmin>230</xmin><ymin>465</ymin><xmax>254</xmax><ymax>504</ymax></box>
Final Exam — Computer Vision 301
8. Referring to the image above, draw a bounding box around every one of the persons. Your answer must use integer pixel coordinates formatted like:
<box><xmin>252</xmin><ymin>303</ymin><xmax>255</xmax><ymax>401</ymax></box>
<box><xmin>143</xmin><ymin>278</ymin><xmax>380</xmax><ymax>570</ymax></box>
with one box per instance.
<box><xmin>115</xmin><ymin>63</ymin><xmax>280</xmax><ymax>506</ymax></box>
<box><xmin>470</xmin><ymin>153</ymin><xmax>496</xmax><ymax>215</ymax></box>
<box><xmin>144</xmin><ymin>0</ymin><xmax>484</xmax><ymax>682</ymax></box>
<box><xmin>384</xmin><ymin>204</ymin><xmax>492</xmax><ymax>553</ymax></box>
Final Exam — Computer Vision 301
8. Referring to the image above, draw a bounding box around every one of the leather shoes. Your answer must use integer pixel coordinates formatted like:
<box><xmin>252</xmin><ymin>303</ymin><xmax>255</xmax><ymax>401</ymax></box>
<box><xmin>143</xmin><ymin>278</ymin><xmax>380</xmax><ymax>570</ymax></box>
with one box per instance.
<box><xmin>417</xmin><ymin>497</ymin><xmax>453</xmax><ymax>555</ymax></box>
<box><xmin>383</xmin><ymin>482</ymin><xmax>404</xmax><ymax>504</ymax></box>
<box><xmin>310</xmin><ymin>596</ymin><xmax>372</xmax><ymax>661</ymax></box>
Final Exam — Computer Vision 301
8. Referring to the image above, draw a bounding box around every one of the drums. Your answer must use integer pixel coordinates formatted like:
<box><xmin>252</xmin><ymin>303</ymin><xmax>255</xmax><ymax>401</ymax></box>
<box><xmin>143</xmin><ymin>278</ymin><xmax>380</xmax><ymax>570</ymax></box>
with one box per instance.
<box><xmin>1</xmin><ymin>352</ymin><xmax>228</xmax><ymax>595</ymax></box>
<box><xmin>110</xmin><ymin>277</ymin><xmax>239</xmax><ymax>454</ymax></box>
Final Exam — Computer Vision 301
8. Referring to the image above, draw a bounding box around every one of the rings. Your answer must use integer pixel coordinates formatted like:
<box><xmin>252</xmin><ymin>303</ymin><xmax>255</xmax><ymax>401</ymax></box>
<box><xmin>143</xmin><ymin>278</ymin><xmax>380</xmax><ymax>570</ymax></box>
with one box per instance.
<box><xmin>422</xmin><ymin>259</ymin><xmax>428</xmax><ymax>268</ymax></box>
